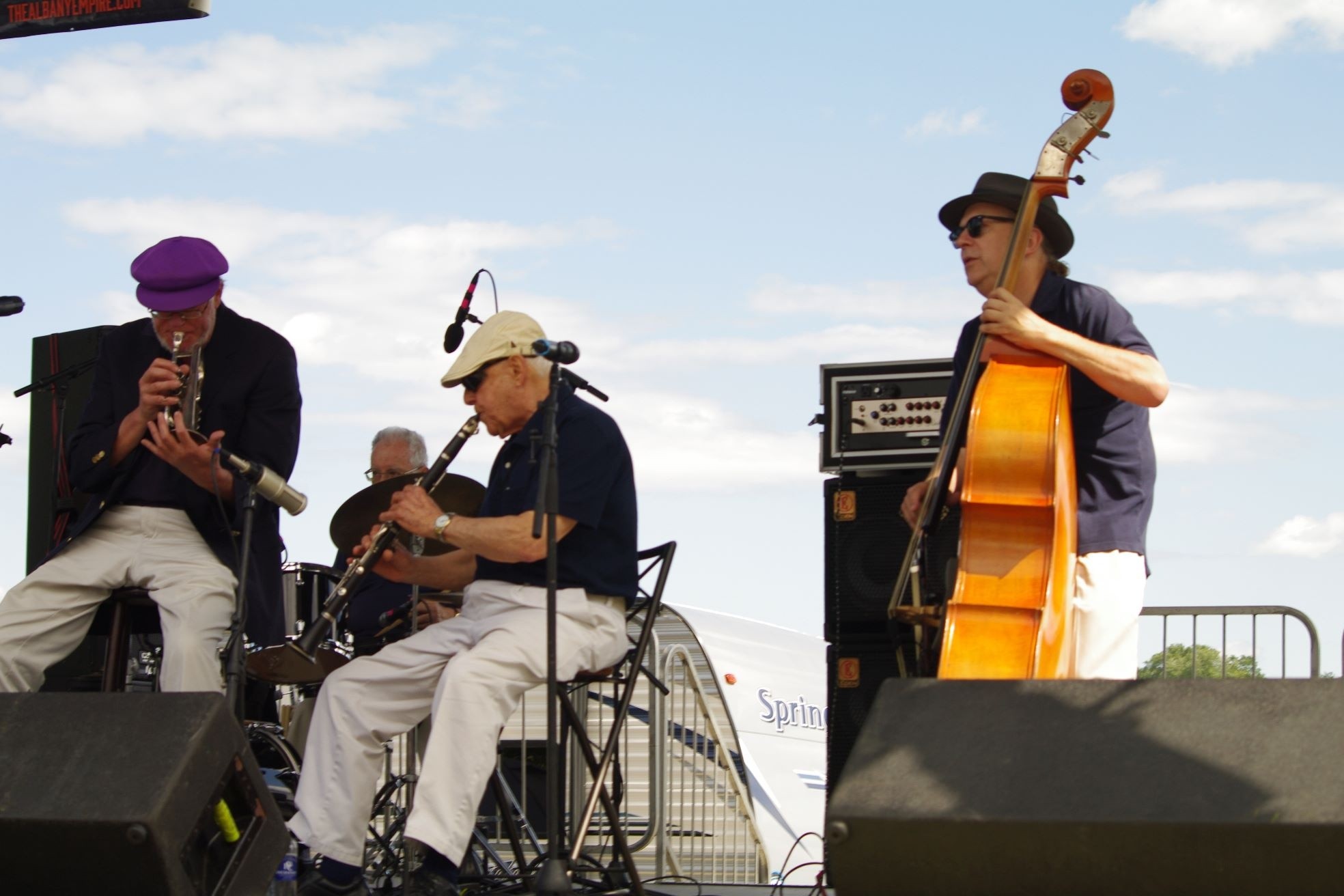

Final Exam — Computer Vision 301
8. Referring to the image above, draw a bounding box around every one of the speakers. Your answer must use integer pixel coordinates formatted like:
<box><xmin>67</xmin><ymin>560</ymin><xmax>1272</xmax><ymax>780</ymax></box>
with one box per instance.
<box><xmin>478</xmin><ymin>741</ymin><xmax>564</xmax><ymax>839</ymax></box>
<box><xmin>26</xmin><ymin>323</ymin><xmax>163</xmax><ymax>691</ymax></box>
<box><xmin>826</xmin><ymin>678</ymin><xmax>1344</xmax><ymax>896</ymax></box>
<box><xmin>1</xmin><ymin>691</ymin><xmax>287</xmax><ymax>896</ymax></box>
<box><xmin>826</xmin><ymin>475</ymin><xmax>959</xmax><ymax>803</ymax></box>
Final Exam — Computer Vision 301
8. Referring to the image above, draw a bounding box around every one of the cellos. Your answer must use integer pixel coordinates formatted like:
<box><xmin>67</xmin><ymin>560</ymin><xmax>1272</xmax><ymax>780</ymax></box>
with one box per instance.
<box><xmin>891</xmin><ymin>64</ymin><xmax>1116</xmax><ymax>681</ymax></box>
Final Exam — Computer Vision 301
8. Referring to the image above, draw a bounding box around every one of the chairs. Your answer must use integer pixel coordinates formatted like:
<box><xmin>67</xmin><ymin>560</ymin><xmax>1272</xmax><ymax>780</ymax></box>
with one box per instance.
<box><xmin>549</xmin><ymin>539</ymin><xmax>678</xmax><ymax>896</ymax></box>
<box><xmin>91</xmin><ymin>588</ymin><xmax>167</xmax><ymax>698</ymax></box>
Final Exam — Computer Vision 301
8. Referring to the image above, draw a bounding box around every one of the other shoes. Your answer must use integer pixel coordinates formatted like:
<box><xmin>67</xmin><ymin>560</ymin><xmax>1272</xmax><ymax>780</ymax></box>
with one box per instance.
<box><xmin>382</xmin><ymin>857</ymin><xmax>462</xmax><ymax>896</ymax></box>
<box><xmin>291</xmin><ymin>872</ymin><xmax>371</xmax><ymax>896</ymax></box>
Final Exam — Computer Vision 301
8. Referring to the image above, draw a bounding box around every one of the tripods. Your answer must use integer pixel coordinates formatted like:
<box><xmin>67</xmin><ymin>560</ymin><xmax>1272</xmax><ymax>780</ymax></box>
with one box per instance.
<box><xmin>369</xmin><ymin>532</ymin><xmax>518</xmax><ymax>887</ymax></box>
<box><xmin>589</xmin><ymin>654</ymin><xmax>669</xmax><ymax>896</ymax></box>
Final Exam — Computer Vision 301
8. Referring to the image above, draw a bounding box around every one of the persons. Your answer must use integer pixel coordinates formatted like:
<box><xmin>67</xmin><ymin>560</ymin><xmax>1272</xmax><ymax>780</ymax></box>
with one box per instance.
<box><xmin>287</xmin><ymin>309</ymin><xmax>638</xmax><ymax>896</ymax></box>
<box><xmin>287</xmin><ymin>429</ymin><xmax>462</xmax><ymax>796</ymax></box>
<box><xmin>1</xmin><ymin>237</ymin><xmax>301</xmax><ymax>692</ymax></box>
<box><xmin>901</xmin><ymin>172</ymin><xmax>1168</xmax><ymax>681</ymax></box>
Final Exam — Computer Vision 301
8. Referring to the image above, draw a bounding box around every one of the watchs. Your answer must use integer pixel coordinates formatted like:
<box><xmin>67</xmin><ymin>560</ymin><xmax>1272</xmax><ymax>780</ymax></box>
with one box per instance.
<box><xmin>435</xmin><ymin>512</ymin><xmax>456</xmax><ymax>543</ymax></box>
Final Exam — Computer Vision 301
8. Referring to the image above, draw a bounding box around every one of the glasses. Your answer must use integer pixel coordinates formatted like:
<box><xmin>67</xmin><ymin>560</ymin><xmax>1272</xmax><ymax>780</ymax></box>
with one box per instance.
<box><xmin>147</xmin><ymin>301</ymin><xmax>207</xmax><ymax>320</ymax></box>
<box><xmin>947</xmin><ymin>213</ymin><xmax>1015</xmax><ymax>248</ymax></box>
<box><xmin>460</xmin><ymin>356</ymin><xmax>509</xmax><ymax>391</ymax></box>
<box><xmin>364</xmin><ymin>466</ymin><xmax>421</xmax><ymax>482</ymax></box>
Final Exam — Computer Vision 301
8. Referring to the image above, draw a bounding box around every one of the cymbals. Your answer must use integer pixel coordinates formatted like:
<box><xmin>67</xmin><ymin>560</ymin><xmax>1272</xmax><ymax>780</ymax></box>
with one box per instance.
<box><xmin>327</xmin><ymin>468</ymin><xmax>487</xmax><ymax>557</ymax></box>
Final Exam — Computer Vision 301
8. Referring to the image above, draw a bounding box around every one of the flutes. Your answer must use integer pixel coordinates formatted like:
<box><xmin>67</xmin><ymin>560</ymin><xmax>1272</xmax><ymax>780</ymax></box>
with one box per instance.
<box><xmin>289</xmin><ymin>414</ymin><xmax>482</xmax><ymax>666</ymax></box>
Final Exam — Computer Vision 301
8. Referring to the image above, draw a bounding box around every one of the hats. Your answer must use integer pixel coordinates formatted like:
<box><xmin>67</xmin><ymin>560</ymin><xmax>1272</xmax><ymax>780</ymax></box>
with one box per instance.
<box><xmin>129</xmin><ymin>236</ymin><xmax>230</xmax><ymax>313</ymax></box>
<box><xmin>938</xmin><ymin>171</ymin><xmax>1074</xmax><ymax>261</ymax></box>
<box><xmin>440</xmin><ymin>310</ymin><xmax>547</xmax><ymax>389</ymax></box>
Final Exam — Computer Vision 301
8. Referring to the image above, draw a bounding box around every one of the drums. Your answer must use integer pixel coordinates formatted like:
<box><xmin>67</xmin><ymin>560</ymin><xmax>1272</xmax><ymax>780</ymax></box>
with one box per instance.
<box><xmin>236</xmin><ymin>553</ymin><xmax>353</xmax><ymax>690</ymax></box>
<box><xmin>231</xmin><ymin>717</ymin><xmax>315</xmax><ymax>793</ymax></box>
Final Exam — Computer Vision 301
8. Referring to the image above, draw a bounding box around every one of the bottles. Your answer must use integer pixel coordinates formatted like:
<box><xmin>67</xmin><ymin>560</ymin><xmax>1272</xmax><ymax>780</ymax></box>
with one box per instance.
<box><xmin>271</xmin><ymin>821</ymin><xmax>298</xmax><ymax>896</ymax></box>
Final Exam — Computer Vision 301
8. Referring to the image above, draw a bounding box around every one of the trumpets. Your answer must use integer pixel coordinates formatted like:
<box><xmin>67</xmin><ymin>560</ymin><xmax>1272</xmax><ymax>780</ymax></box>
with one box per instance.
<box><xmin>161</xmin><ymin>329</ymin><xmax>211</xmax><ymax>445</ymax></box>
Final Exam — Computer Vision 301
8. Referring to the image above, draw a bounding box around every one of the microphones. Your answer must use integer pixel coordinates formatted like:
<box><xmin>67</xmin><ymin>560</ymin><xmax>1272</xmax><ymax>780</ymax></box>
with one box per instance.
<box><xmin>532</xmin><ymin>339</ymin><xmax>580</xmax><ymax>364</ymax></box>
<box><xmin>379</xmin><ymin>598</ymin><xmax>413</xmax><ymax>628</ymax></box>
<box><xmin>444</xmin><ymin>273</ymin><xmax>478</xmax><ymax>354</ymax></box>
<box><xmin>0</xmin><ymin>296</ymin><xmax>25</xmax><ymax>317</ymax></box>
<box><xmin>217</xmin><ymin>449</ymin><xmax>308</xmax><ymax>515</ymax></box>
